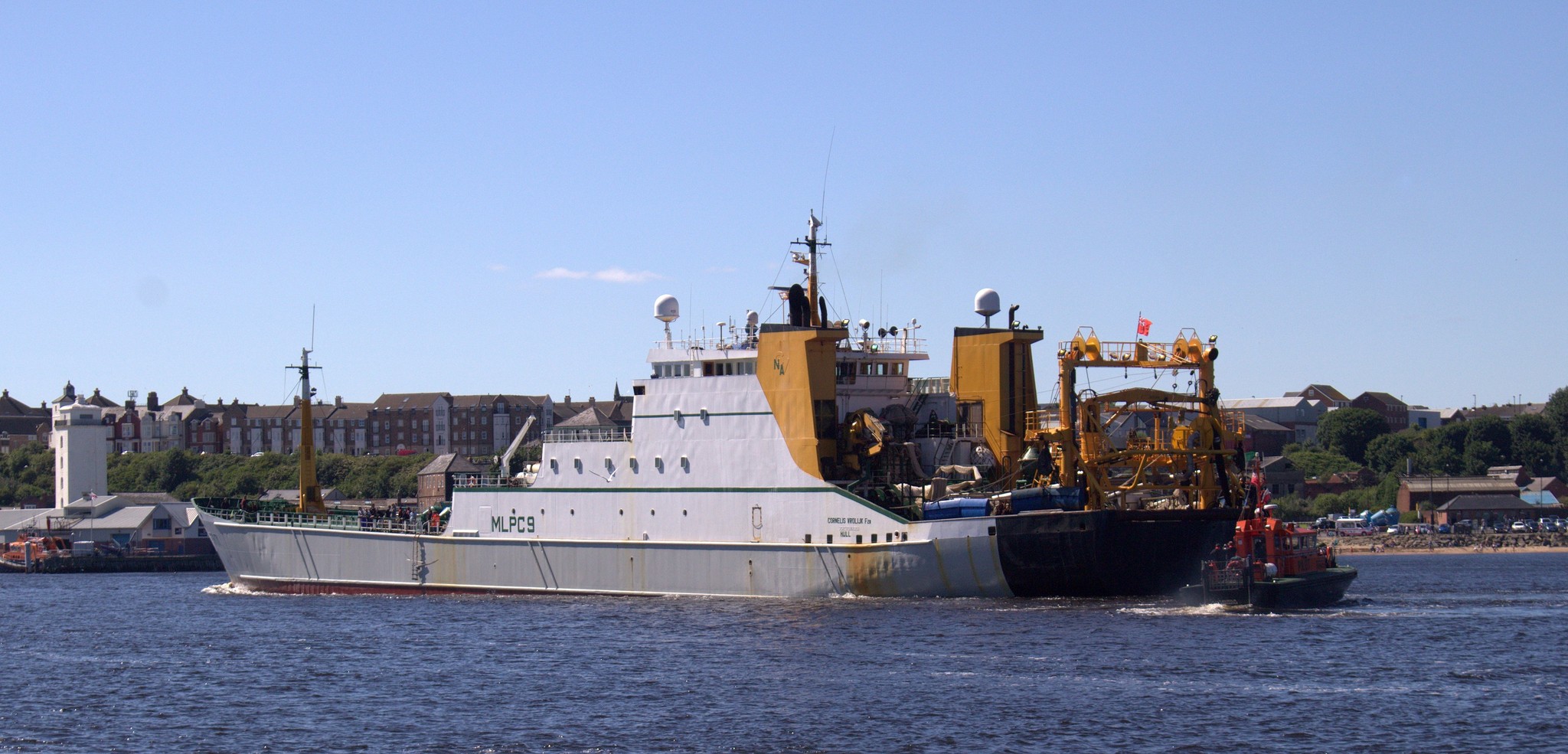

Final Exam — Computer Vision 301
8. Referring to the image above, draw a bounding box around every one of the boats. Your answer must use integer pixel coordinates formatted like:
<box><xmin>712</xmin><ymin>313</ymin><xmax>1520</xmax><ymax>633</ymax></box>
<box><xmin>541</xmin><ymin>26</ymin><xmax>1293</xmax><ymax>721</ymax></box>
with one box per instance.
<box><xmin>0</xmin><ymin>490</ymin><xmax>104</xmax><ymax>571</ymax></box>
<box><xmin>1179</xmin><ymin>450</ymin><xmax>1359</xmax><ymax>611</ymax></box>
<box><xmin>190</xmin><ymin>100</ymin><xmax>1250</xmax><ymax>601</ymax></box>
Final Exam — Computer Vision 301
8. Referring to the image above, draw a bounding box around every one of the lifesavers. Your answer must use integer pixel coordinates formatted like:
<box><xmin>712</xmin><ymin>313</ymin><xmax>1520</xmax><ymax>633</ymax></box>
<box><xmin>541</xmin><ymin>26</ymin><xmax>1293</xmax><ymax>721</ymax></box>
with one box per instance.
<box><xmin>468</xmin><ymin>476</ymin><xmax>475</xmax><ymax>486</ymax></box>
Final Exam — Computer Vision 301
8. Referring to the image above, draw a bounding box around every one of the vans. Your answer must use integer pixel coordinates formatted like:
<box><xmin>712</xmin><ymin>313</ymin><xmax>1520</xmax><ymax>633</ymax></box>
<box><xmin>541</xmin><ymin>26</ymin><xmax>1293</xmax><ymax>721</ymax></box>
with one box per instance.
<box><xmin>1335</xmin><ymin>518</ymin><xmax>1372</xmax><ymax>537</ymax></box>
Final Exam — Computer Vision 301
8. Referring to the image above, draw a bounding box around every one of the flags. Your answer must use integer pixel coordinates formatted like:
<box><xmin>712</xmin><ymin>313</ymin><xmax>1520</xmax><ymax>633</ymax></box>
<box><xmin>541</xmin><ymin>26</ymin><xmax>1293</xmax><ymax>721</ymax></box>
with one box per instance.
<box><xmin>91</xmin><ymin>492</ymin><xmax>96</xmax><ymax>500</ymax></box>
<box><xmin>1138</xmin><ymin>316</ymin><xmax>1152</xmax><ymax>336</ymax></box>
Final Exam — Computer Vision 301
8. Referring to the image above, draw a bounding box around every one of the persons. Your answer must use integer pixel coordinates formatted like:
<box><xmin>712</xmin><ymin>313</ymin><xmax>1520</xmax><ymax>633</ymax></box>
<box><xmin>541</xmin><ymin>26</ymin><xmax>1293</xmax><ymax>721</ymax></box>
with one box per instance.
<box><xmin>1428</xmin><ymin>539</ymin><xmax>1432</xmax><ymax>551</ymax></box>
<box><xmin>221</xmin><ymin>495</ymin><xmax>281</xmax><ymax>523</ymax></box>
<box><xmin>1473</xmin><ymin>517</ymin><xmax>1486</xmax><ymax>535</ymax></box>
<box><xmin>1369</xmin><ymin>521</ymin><xmax>1433</xmax><ymax>536</ymax></box>
<box><xmin>357</xmin><ymin>503</ymin><xmax>440</xmax><ymax>535</ymax></box>
<box><xmin>1503</xmin><ymin>514</ymin><xmax>1567</xmax><ymax>534</ymax></box>
<box><xmin>1370</xmin><ymin>539</ymin><xmax>1395</xmax><ymax>552</ymax></box>
<box><xmin>1296</xmin><ymin>522</ymin><xmax>1338</xmax><ymax>538</ymax></box>
<box><xmin>1209</xmin><ymin>541</ymin><xmax>1237</xmax><ymax>582</ymax></box>
<box><xmin>1339</xmin><ymin>548</ymin><xmax>1342</xmax><ymax>554</ymax></box>
<box><xmin>1481</xmin><ymin>539</ymin><xmax>1515</xmax><ymax>552</ymax></box>
<box><xmin>1350</xmin><ymin>547</ymin><xmax>1353</xmax><ymax>556</ymax></box>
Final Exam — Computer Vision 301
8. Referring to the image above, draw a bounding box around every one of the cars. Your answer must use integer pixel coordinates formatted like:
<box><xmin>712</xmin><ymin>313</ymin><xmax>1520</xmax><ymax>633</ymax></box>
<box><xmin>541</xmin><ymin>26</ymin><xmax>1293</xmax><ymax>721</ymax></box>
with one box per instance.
<box><xmin>1492</xmin><ymin>523</ymin><xmax>1504</xmax><ymax>533</ymax></box>
<box><xmin>1512</xmin><ymin>515</ymin><xmax>1568</xmax><ymax>532</ymax></box>
<box><xmin>1387</xmin><ymin>524</ymin><xmax>1402</xmax><ymax>534</ymax></box>
<box><xmin>1439</xmin><ymin>519</ymin><xmax>1472</xmax><ymax>534</ymax></box>
<box><xmin>121</xmin><ymin>450</ymin><xmax>132</xmax><ymax>455</ymax></box>
<box><xmin>1316</xmin><ymin>517</ymin><xmax>1335</xmax><ymax>529</ymax></box>
<box><xmin>250</xmin><ymin>452</ymin><xmax>265</xmax><ymax>456</ymax></box>
<box><xmin>1415</xmin><ymin>523</ymin><xmax>1430</xmax><ymax>534</ymax></box>
